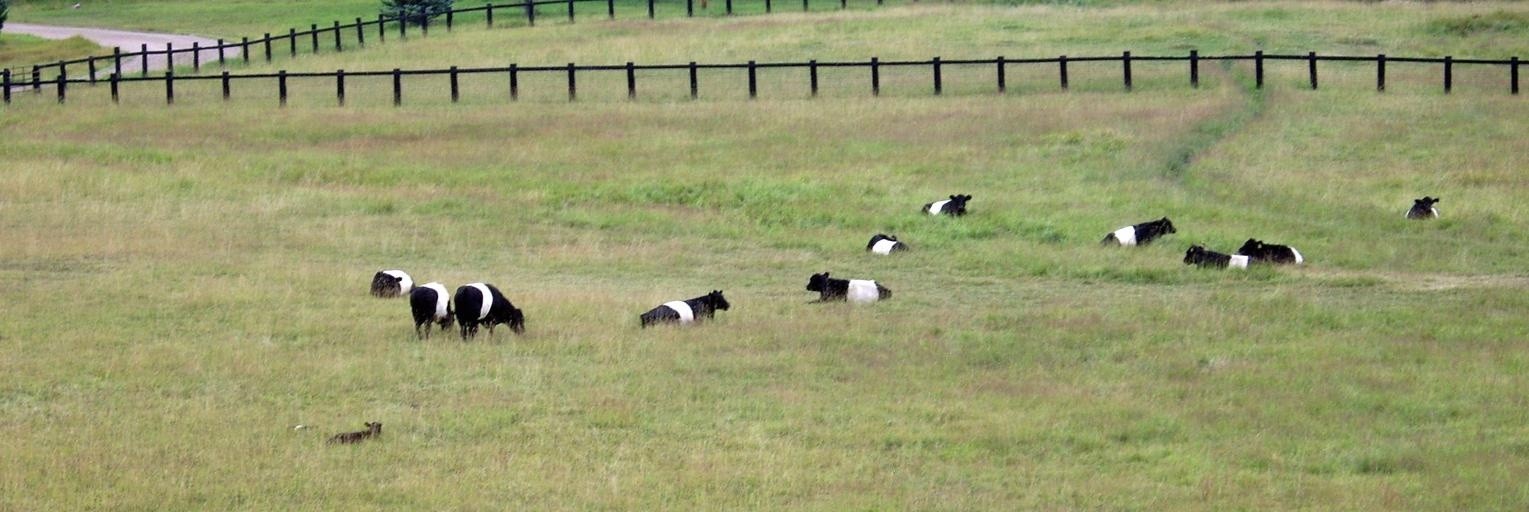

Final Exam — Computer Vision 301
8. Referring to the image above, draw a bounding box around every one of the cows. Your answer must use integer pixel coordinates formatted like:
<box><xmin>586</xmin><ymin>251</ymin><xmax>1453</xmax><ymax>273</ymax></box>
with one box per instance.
<box><xmin>327</xmin><ymin>421</ymin><xmax>382</xmax><ymax>445</ymax></box>
<box><xmin>453</xmin><ymin>282</ymin><xmax>525</xmax><ymax>343</ymax></box>
<box><xmin>1237</xmin><ymin>238</ymin><xmax>1305</xmax><ymax>265</ymax></box>
<box><xmin>409</xmin><ymin>281</ymin><xmax>455</xmax><ymax>341</ymax></box>
<box><xmin>921</xmin><ymin>194</ymin><xmax>972</xmax><ymax>219</ymax></box>
<box><xmin>806</xmin><ymin>271</ymin><xmax>892</xmax><ymax>304</ymax></box>
<box><xmin>1099</xmin><ymin>216</ymin><xmax>1177</xmax><ymax>248</ymax></box>
<box><xmin>370</xmin><ymin>269</ymin><xmax>416</xmax><ymax>299</ymax></box>
<box><xmin>1183</xmin><ymin>244</ymin><xmax>1256</xmax><ymax>274</ymax></box>
<box><xmin>640</xmin><ymin>289</ymin><xmax>730</xmax><ymax>330</ymax></box>
<box><xmin>1404</xmin><ymin>196</ymin><xmax>1440</xmax><ymax>220</ymax></box>
<box><xmin>866</xmin><ymin>233</ymin><xmax>909</xmax><ymax>256</ymax></box>
